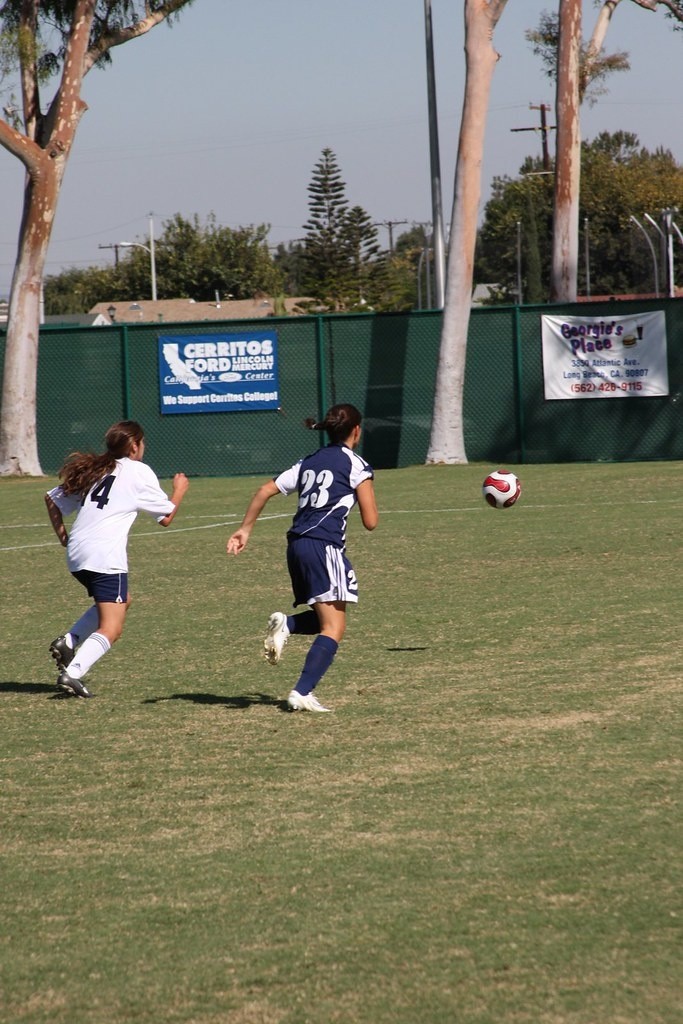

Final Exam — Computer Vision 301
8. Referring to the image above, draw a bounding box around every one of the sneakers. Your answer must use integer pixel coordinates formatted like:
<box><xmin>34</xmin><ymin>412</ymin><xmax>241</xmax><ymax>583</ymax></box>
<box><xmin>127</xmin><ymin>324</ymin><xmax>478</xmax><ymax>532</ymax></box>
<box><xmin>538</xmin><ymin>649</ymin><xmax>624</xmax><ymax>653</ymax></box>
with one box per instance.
<box><xmin>263</xmin><ymin>613</ymin><xmax>291</xmax><ymax>665</ymax></box>
<box><xmin>56</xmin><ymin>673</ymin><xmax>93</xmax><ymax>698</ymax></box>
<box><xmin>287</xmin><ymin>688</ymin><xmax>332</xmax><ymax>712</ymax></box>
<box><xmin>49</xmin><ymin>635</ymin><xmax>75</xmax><ymax>672</ymax></box>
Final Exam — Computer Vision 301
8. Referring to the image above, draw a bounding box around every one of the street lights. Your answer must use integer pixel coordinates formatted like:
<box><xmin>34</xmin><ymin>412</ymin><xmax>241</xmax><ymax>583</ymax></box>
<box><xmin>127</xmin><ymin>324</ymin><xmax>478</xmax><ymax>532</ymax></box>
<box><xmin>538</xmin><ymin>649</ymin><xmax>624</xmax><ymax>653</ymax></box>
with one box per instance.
<box><xmin>418</xmin><ymin>247</ymin><xmax>434</xmax><ymax>311</ymax></box>
<box><xmin>629</xmin><ymin>214</ymin><xmax>659</xmax><ymax>300</ymax></box>
<box><xmin>119</xmin><ymin>213</ymin><xmax>159</xmax><ymax>300</ymax></box>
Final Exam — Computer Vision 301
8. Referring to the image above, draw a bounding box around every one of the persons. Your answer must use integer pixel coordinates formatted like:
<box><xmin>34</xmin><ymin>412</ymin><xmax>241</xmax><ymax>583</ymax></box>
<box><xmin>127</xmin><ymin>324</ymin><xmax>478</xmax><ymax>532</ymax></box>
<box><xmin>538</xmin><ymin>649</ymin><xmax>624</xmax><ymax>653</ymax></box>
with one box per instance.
<box><xmin>45</xmin><ymin>421</ymin><xmax>189</xmax><ymax>698</ymax></box>
<box><xmin>226</xmin><ymin>404</ymin><xmax>378</xmax><ymax>713</ymax></box>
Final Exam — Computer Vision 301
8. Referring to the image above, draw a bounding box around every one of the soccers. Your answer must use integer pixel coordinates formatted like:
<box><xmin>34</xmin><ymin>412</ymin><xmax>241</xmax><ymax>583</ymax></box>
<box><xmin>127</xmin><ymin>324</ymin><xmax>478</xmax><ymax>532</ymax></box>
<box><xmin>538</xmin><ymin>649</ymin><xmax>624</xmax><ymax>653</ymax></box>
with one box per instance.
<box><xmin>482</xmin><ymin>469</ymin><xmax>522</xmax><ymax>510</ymax></box>
